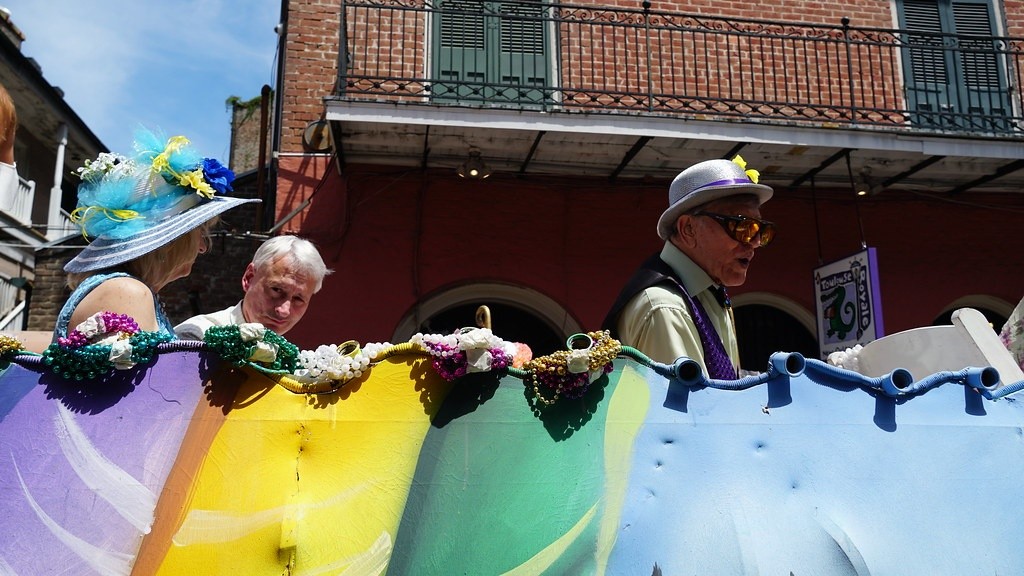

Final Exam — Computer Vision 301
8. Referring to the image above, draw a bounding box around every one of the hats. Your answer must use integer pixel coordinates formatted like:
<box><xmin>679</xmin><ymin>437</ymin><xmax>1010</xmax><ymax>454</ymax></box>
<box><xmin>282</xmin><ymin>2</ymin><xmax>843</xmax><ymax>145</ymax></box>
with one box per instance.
<box><xmin>63</xmin><ymin>122</ymin><xmax>262</xmax><ymax>273</ymax></box>
<box><xmin>657</xmin><ymin>154</ymin><xmax>774</xmax><ymax>241</ymax></box>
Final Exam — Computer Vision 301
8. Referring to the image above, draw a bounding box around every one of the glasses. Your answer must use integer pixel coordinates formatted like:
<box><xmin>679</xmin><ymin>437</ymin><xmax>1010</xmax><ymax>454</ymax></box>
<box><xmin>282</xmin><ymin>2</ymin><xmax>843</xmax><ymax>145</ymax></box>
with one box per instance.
<box><xmin>688</xmin><ymin>211</ymin><xmax>776</xmax><ymax>248</ymax></box>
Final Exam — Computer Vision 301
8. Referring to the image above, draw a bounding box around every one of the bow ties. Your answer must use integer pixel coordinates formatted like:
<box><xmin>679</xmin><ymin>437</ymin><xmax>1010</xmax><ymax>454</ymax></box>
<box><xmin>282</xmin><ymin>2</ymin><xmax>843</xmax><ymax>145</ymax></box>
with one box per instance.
<box><xmin>710</xmin><ymin>285</ymin><xmax>731</xmax><ymax>309</ymax></box>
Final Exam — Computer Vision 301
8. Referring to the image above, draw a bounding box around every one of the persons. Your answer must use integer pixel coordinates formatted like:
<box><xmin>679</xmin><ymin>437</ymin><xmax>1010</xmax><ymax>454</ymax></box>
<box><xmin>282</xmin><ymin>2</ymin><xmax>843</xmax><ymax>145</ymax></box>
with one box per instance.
<box><xmin>600</xmin><ymin>159</ymin><xmax>774</xmax><ymax>379</ymax></box>
<box><xmin>54</xmin><ymin>128</ymin><xmax>263</xmax><ymax>343</ymax></box>
<box><xmin>172</xmin><ymin>235</ymin><xmax>332</xmax><ymax>339</ymax></box>
<box><xmin>0</xmin><ymin>83</ymin><xmax>20</xmax><ymax>210</ymax></box>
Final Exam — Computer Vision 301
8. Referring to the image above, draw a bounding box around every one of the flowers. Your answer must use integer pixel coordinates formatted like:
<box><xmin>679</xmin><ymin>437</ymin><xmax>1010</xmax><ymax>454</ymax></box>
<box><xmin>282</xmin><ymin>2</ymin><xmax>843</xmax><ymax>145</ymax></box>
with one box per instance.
<box><xmin>70</xmin><ymin>136</ymin><xmax>236</xmax><ymax>200</ymax></box>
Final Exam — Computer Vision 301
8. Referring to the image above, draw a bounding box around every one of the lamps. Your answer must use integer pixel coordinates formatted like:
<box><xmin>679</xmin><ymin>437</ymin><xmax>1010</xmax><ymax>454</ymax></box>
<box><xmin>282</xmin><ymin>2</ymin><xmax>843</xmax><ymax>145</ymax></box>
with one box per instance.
<box><xmin>455</xmin><ymin>151</ymin><xmax>491</xmax><ymax>180</ymax></box>
<box><xmin>852</xmin><ymin>168</ymin><xmax>871</xmax><ymax>196</ymax></box>
